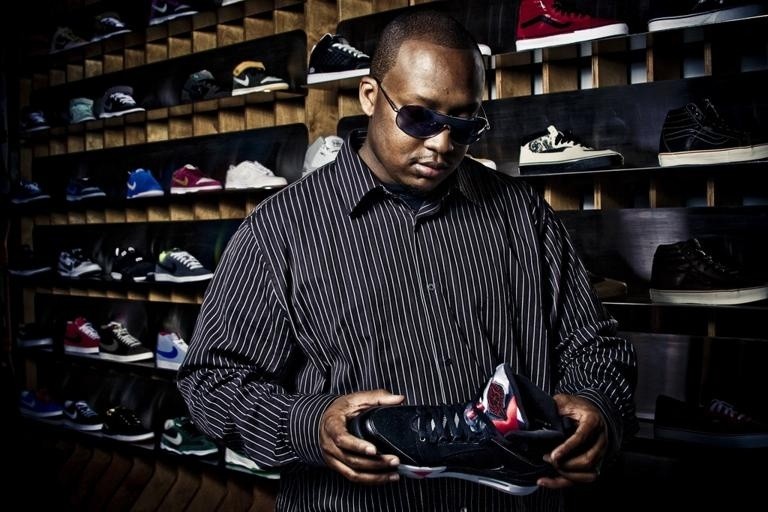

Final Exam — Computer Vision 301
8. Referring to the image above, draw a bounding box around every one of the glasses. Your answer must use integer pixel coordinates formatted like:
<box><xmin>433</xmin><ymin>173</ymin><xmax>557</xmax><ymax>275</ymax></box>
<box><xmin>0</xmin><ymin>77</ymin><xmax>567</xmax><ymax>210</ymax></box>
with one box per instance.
<box><xmin>376</xmin><ymin>76</ymin><xmax>491</xmax><ymax>147</ymax></box>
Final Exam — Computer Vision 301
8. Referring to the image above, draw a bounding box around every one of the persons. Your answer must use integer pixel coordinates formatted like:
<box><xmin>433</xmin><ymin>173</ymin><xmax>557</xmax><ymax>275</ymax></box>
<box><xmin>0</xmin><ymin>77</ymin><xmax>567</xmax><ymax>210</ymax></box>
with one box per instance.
<box><xmin>177</xmin><ymin>13</ymin><xmax>638</xmax><ymax>510</ymax></box>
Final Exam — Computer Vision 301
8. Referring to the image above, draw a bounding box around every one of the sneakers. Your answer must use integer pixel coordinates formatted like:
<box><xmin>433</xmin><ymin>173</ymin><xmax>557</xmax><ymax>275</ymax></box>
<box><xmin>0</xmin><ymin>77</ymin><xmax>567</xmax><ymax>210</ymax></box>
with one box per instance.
<box><xmin>9</xmin><ymin>242</ymin><xmax>214</xmax><ymax>283</ymax></box>
<box><xmin>301</xmin><ymin>134</ymin><xmax>346</xmax><ymax>178</ymax></box>
<box><xmin>16</xmin><ymin>319</ymin><xmax>189</xmax><ymax>370</ymax></box>
<box><xmin>585</xmin><ymin>269</ymin><xmax>629</xmax><ymax>300</ymax></box>
<box><xmin>231</xmin><ymin>61</ymin><xmax>290</xmax><ymax>97</ymax></box>
<box><xmin>516</xmin><ymin>125</ymin><xmax>624</xmax><ymax>175</ymax></box>
<box><xmin>19</xmin><ymin>390</ymin><xmax>265</xmax><ymax>472</ymax></box>
<box><xmin>48</xmin><ymin>14</ymin><xmax>131</xmax><ymax>56</ymax></box>
<box><xmin>649</xmin><ymin>237</ymin><xmax>767</xmax><ymax>305</ymax></box>
<box><xmin>657</xmin><ymin>97</ymin><xmax>768</xmax><ymax>167</ymax></box>
<box><xmin>13</xmin><ymin>161</ymin><xmax>287</xmax><ymax>205</ymax></box>
<box><xmin>514</xmin><ymin>0</ymin><xmax>630</xmax><ymax>54</ymax></box>
<box><xmin>654</xmin><ymin>394</ymin><xmax>767</xmax><ymax>449</ymax></box>
<box><xmin>347</xmin><ymin>363</ymin><xmax>567</xmax><ymax>497</ymax></box>
<box><xmin>18</xmin><ymin>85</ymin><xmax>145</xmax><ymax>134</ymax></box>
<box><xmin>181</xmin><ymin>68</ymin><xmax>230</xmax><ymax>100</ymax></box>
<box><xmin>148</xmin><ymin>1</ymin><xmax>197</xmax><ymax>26</ymax></box>
<box><xmin>646</xmin><ymin>0</ymin><xmax>762</xmax><ymax>33</ymax></box>
<box><xmin>305</xmin><ymin>32</ymin><xmax>373</xmax><ymax>90</ymax></box>
